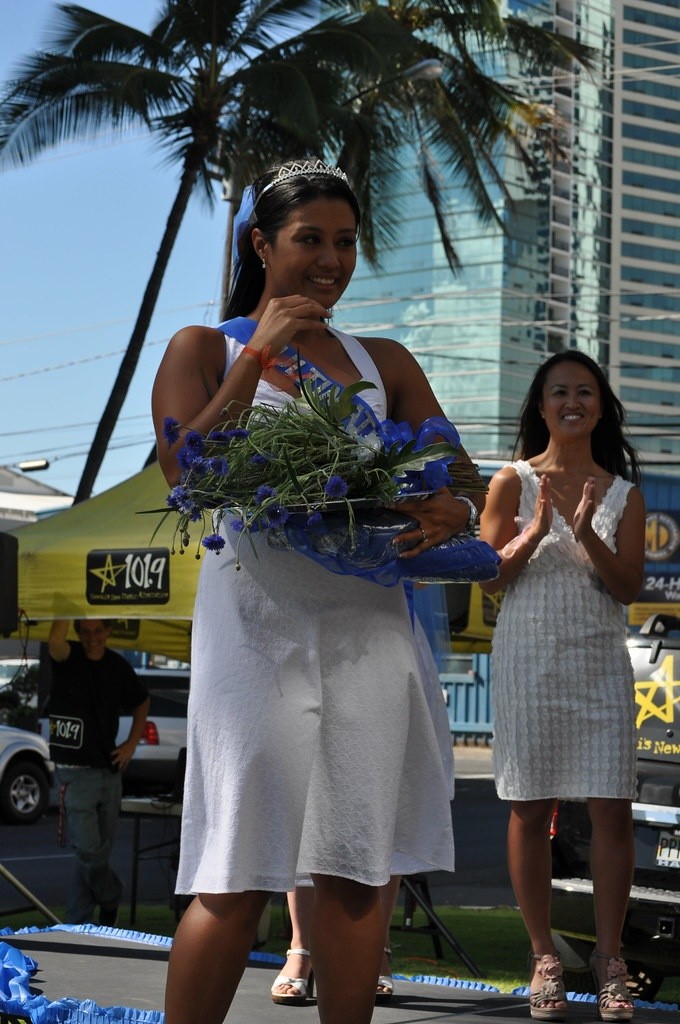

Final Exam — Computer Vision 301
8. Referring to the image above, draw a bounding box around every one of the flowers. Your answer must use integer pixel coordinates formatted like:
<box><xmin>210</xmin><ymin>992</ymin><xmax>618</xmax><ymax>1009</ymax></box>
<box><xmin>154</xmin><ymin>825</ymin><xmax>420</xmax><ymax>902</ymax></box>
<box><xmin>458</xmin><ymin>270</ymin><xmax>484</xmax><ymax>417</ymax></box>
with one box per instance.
<box><xmin>136</xmin><ymin>337</ymin><xmax>490</xmax><ymax>570</ymax></box>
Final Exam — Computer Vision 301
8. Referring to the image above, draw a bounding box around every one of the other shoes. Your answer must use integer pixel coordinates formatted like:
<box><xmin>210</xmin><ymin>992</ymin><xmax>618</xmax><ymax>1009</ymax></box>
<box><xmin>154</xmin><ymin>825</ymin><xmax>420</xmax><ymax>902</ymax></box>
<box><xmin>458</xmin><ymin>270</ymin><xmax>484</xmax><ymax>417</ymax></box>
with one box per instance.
<box><xmin>99</xmin><ymin>881</ymin><xmax>124</xmax><ymax>929</ymax></box>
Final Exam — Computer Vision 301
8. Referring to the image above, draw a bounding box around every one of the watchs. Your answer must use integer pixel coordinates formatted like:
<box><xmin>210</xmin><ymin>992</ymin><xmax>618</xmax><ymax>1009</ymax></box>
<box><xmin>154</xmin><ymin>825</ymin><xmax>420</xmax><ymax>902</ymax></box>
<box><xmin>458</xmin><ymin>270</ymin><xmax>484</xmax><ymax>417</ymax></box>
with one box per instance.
<box><xmin>453</xmin><ymin>495</ymin><xmax>478</xmax><ymax>536</ymax></box>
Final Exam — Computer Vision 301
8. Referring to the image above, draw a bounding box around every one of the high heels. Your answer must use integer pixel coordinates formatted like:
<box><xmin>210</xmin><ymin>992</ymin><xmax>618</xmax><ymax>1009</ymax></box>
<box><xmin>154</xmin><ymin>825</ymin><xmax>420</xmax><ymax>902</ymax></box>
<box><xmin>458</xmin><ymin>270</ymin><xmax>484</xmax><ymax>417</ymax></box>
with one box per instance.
<box><xmin>271</xmin><ymin>948</ymin><xmax>313</xmax><ymax>1004</ymax></box>
<box><xmin>375</xmin><ymin>947</ymin><xmax>394</xmax><ymax>1005</ymax></box>
<box><xmin>588</xmin><ymin>947</ymin><xmax>634</xmax><ymax>1021</ymax></box>
<box><xmin>529</xmin><ymin>950</ymin><xmax>567</xmax><ymax>1019</ymax></box>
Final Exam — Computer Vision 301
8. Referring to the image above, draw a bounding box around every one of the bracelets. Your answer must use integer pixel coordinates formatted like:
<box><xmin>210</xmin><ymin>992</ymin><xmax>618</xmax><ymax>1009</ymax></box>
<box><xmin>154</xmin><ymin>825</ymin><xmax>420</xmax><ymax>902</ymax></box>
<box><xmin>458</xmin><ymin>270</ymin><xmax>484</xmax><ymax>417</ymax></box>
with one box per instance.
<box><xmin>242</xmin><ymin>344</ymin><xmax>297</xmax><ymax>392</ymax></box>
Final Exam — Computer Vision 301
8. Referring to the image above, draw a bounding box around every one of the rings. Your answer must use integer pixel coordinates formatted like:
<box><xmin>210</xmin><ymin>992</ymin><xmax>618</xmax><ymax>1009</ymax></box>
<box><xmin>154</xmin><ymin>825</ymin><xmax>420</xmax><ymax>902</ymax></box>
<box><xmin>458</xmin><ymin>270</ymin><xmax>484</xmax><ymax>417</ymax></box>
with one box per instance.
<box><xmin>419</xmin><ymin>526</ymin><xmax>429</xmax><ymax>542</ymax></box>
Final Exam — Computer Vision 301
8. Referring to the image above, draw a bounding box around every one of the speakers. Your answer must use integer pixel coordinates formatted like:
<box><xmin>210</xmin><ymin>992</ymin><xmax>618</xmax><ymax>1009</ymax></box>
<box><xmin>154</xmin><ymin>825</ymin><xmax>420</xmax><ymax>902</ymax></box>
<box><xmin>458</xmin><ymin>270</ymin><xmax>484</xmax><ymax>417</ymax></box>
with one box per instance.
<box><xmin>0</xmin><ymin>531</ymin><xmax>20</xmax><ymax>638</ymax></box>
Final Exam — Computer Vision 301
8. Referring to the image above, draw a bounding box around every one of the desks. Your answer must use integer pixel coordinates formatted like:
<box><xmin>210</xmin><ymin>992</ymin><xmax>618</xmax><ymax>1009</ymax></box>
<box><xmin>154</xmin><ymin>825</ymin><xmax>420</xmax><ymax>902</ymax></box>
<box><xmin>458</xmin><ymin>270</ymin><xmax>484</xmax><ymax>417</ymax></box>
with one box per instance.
<box><xmin>120</xmin><ymin>791</ymin><xmax>453</xmax><ymax>960</ymax></box>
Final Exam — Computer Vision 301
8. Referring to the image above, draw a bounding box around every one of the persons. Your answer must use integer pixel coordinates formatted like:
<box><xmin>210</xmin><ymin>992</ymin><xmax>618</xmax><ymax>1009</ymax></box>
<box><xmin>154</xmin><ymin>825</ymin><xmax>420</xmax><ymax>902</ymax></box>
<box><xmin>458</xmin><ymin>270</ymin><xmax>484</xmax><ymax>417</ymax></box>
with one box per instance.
<box><xmin>47</xmin><ymin>619</ymin><xmax>149</xmax><ymax>927</ymax></box>
<box><xmin>152</xmin><ymin>158</ymin><xmax>486</xmax><ymax>1024</ymax></box>
<box><xmin>479</xmin><ymin>349</ymin><xmax>645</xmax><ymax>1019</ymax></box>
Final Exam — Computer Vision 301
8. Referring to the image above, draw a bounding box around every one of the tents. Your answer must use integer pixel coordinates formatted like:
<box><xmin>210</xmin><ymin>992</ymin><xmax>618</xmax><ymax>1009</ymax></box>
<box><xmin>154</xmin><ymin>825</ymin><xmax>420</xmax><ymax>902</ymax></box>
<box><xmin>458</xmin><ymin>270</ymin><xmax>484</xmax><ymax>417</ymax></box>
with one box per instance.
<box><xmin>0</xmin><ymin>458</ymin><xmax>508</xmax><ymax>665</ymax></box>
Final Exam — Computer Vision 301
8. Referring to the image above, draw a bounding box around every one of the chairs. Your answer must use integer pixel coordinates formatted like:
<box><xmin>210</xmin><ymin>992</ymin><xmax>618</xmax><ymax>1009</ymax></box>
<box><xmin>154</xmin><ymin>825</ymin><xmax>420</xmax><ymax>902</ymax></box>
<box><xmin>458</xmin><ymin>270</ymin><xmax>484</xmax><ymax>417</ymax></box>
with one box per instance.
<box><xmin>639</xmin><ymin>783</ymin><xmax>678</xmax><ymax>805</ymax></box>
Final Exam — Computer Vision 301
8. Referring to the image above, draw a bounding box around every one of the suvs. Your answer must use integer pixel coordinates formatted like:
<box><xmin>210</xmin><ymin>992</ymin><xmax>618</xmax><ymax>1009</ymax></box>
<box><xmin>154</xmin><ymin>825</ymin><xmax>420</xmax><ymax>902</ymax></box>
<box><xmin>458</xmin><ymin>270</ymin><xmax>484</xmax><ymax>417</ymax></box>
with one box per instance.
<box><xmin>550</xmin><ymin>614</ymin><xmax>680</xmax><ymax>973</ymax></box>
<box><xmin>0</xmin><ymin>659</ymin><xmax>190</xmax><ymax>824</ymax></box>
